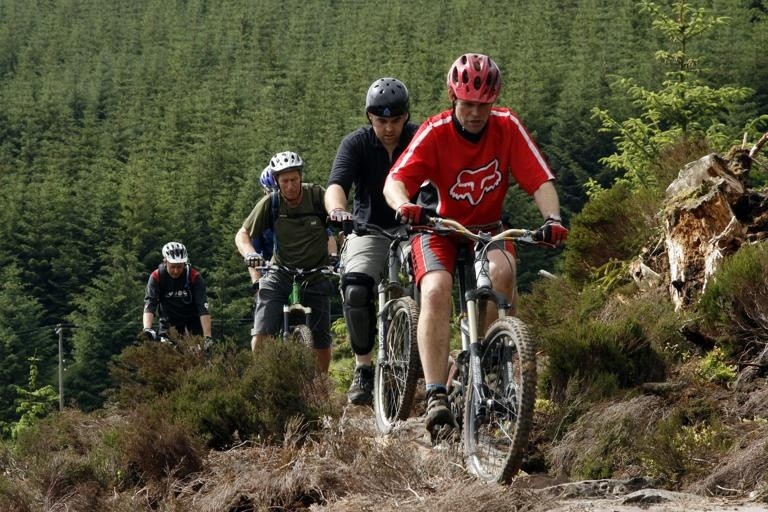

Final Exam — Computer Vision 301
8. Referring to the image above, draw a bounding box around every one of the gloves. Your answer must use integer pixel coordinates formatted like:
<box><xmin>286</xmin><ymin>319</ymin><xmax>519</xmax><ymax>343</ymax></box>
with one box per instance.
<box><xmin>329</xmin><ymin>207</ymin><xmax>360</xmax><ymax>235</ymax></box>
<box><xmin>395</xmin><ymin>202</ymin><xmax>429</xmax><ymax>228</ymax></box>
<box><xmin>244</xmin><ymin>253</ymin><xmax>265</xmax><ymax>269</ymax></box>
<box><xmin>532</xmin><ymin>221</ymin><xmax>569</xmax><ymax>247</ymax></box>
<box><xmin>141</xmin><ymin>327</ymin><xmax>157</xmax><ymax>339</ymax></box>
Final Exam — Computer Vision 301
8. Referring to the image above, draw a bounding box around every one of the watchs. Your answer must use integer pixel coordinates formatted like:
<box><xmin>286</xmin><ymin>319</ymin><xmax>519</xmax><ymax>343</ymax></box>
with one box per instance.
<box><xmin>330</xmin><ymin>251</ymin><xmax>338</xmax><ymax>258</ymax></box>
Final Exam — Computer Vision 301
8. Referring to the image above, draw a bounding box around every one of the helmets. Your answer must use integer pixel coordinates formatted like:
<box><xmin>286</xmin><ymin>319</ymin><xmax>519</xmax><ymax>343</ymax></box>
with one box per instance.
<box><xmin>446</xmin><ymin>53</ymin><xmax>502</xmax><ymax>105</ymax></box>
<box><xmin>260</xmin><ymin>165</ymin><xmax>277</xmax><ymax>192</ymax></box>
<box><xmin>365</xmin><ymin>77</ymin><xmax>411</xmax><ymax>117</ymax></box>
<box><xmin>162</xmin><ymin>241</ymin><xmax>189</xmax><ymax>264</ymax></box>
<box><xmin>271</xmin><ymin>150</ymin><xmax>303</xmax><ymax>175</ymax></box>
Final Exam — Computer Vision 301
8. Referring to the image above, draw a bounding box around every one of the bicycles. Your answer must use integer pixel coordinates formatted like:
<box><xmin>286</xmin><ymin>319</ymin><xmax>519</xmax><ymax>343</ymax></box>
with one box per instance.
<box><xmin>324</xmin><ymin>215</ymin><xmax>416</xmax><ymax>433</ymax></box>
<box><xmin>139</xmin><ymin>329</ymin><xmax>215</xmax><ymax>360</ymax></box>
<box><xmin>254</xmin><ymin>257</ymin><xmax>342</xmax><ymax>384</ymax></box>
<box><xmin>396</xmin><ymin>209</ymin><xmax>568</xmax><ymax>487</ymax></box>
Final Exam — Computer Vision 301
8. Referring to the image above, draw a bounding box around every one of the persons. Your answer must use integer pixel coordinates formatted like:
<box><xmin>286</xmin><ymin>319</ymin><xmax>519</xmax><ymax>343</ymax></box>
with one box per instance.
<box><xmin>379</xmin><ymin>52</ymin><xmax>570</xmax><ymax>477</ymax></box>
<box><xmin>142</xmin><ymin>241</ymin><xmax>213</xmax><ymax>346</ymax></box>
<box><xmin>243</xmin><ymin>166</ymin><xmax>339</xmax><ymax>288</ymax></box>
<box><xmin>319</xmin><ymin>77</ymin><xmax>427</xmax><ymax>408</ymax></box>
<box><xmin>233</xmin><ymin>150</ymin><xmax>333</xmax><ymax>379</ymax></box>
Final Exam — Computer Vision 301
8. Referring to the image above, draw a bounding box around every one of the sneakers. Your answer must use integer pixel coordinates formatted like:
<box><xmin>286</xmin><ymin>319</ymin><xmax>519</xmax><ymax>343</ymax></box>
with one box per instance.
<box><xmin>521</xmin><ymin>440</ymin><xmax>546</xmax><ymax>473</ymax></box>
<box><xmin>348</xmin><ymin>360</ymin><xmax>375</xmax><ymax>405</ymax></box>
<box><xmin>424</xmin><ymin>385</ymin><xmax>454</xmax><ymax>442</ymax></box>
